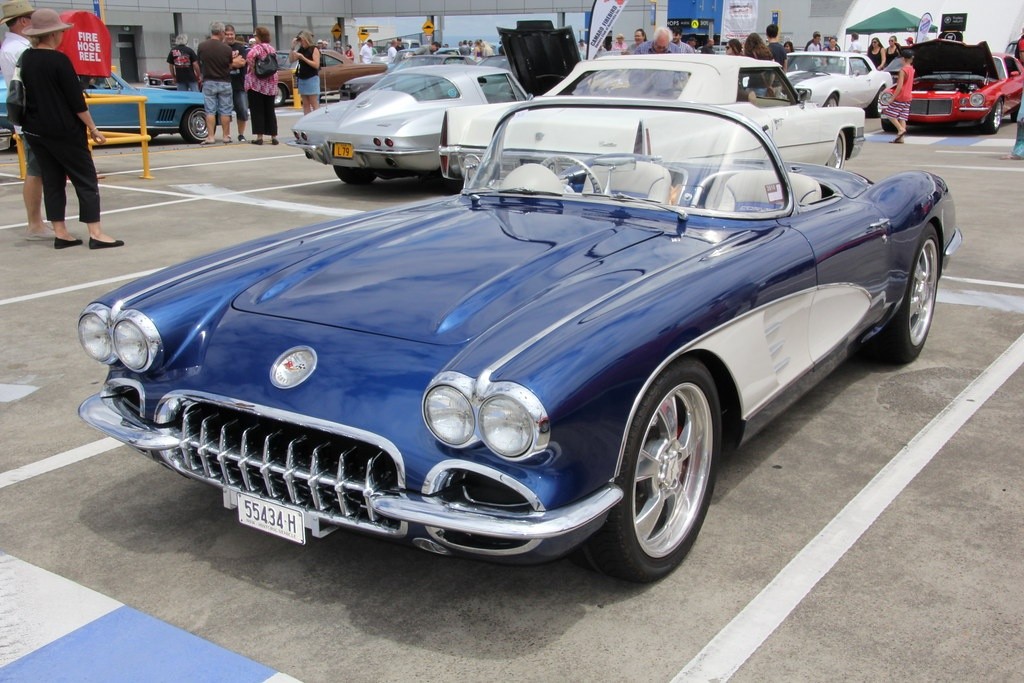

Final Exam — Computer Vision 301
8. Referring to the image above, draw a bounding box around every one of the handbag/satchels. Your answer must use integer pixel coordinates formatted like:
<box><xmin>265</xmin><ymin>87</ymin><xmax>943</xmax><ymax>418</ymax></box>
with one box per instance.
<box><xmin>6</xmin><ymin>49</ymin><xmax>30</xmax><ymax>126</ymax></box>
<box><xmin>254</xmin><ymin>43</ymin><xmax>278</xmax><ymax>79</ymax></box>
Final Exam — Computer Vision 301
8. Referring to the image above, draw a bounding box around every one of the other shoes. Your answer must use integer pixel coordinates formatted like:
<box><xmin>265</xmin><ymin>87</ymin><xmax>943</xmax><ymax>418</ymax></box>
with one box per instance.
<box><xmin>238</xmin><ymin>135</ymin><xmax>246</xmax><ymax>142</ymax></box>
<box><xmin>272</xmin><ymin>139</ymin><xmax>278</xmax><ymax>145</ymax></box>
<box><xmin>1001</xmin><ymin>153</ymin><xmax>1022</xmax><ymax>160</ymax></box>
<box><xmin>25</xmin><ymin>226</ymin><xmax>55</xmax><ymax>240</ymax></box>
<box><xmin>889</xmin><ymin>138</ymin><xmax>904</xmax><ymax>143</ymax></box>
<box><xmin>54</xmin><ymin>237</ymin><xmax>83</xmax><ymax>249</ymax></box>
<box><xmin>88</xmin><ymin>237</ymin><xmax>124</xmax><ymax>249</ymax></box>
<box><xmin>894</xmin><ymin>129</ymin><xmax>906</xmax><ymax>142</ymax></box>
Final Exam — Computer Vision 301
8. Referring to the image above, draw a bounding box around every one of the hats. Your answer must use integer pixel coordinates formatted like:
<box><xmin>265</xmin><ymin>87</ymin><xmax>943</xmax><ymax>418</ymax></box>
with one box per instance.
<box><xmin>0</xmin><ymin>0</ymin><xmax>36</xmax><ymax>25</ymax></box>
<box><xmin>905</xmin><ymin>37</ymin><xmax>913</xmax><ymax>43</ymax></box>
<box><xmin>21</xmin><ymin>7</ymin><xmax>74</xmax><ymax>37</ymax></box>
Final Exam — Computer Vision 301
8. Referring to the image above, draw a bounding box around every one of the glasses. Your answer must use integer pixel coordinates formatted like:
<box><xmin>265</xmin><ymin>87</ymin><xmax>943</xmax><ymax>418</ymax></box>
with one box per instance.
<box><xmin>253</xmin><ymin>33</ymin><xmax>257</xmax><ymax>37</ymax></box>
<box><xmin>295</xmin><ymin>37</ymin><xmax>303</xmax><ymax>42</ymax></box>
<box><xmin>725</xmin><ymin>46</ymin><xmax>731</xmax><ymax>50</ymax></box>
<box><xmin>223</xmin><ymin>31</ymin><xmax>227</xmax><ymax>36</ymax></box>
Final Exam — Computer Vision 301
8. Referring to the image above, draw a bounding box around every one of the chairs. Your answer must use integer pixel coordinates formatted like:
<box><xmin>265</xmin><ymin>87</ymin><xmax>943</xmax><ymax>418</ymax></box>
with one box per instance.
<box><xmin>737</xmin><ymin>89</ymin><xmax>758</xmax><ymax>107</ymax></box>
<box><xmin>580</xmin><ymin>161</ymin><xmax>672</xmax><ymax>204</ymax></box>
<box><xmin>716</xmin><ymin>169</ymin><xmax>822</xmax><ymax>212</ymax></box>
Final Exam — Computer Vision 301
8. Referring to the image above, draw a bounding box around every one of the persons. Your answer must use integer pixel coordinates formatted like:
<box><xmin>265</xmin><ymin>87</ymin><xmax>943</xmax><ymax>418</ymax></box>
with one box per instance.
<box><xmin>20</xmin><ymin>8</ymin><xmax>127</xmax><ymax>249</ymax></box>
<box><xmin>167</xmin><ymin>20</ymin><xmax>504</xmax><ymax>145</ymax></box>
<box><xmin>580</xmin><ymin>25</ymin><xmax>1024</xmax><ymax>141</ymax></box>
<box><xmin>1</xmin><ymin>0</ymin><xmax>61</xmax><ymax>240</ymax></box>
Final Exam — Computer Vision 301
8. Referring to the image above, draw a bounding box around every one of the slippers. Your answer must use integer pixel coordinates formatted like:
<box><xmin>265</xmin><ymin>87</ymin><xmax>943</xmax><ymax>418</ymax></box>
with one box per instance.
<box><xmin>223</xmin><ymin>136</ymin><xmax>233</xmax><ymax>144</ymax></box>
<box><xmin>201</xmin><ymin>141</ymin><xmax>215</xmax><ymax>145</ymax></box>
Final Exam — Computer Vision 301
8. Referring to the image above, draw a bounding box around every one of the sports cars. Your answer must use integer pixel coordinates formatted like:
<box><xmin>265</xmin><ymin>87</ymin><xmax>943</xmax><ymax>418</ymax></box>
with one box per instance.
<box><xmin>73</xmin><ymin>97</ymin><xmax>961</xmax><ymax>587</ymax></box>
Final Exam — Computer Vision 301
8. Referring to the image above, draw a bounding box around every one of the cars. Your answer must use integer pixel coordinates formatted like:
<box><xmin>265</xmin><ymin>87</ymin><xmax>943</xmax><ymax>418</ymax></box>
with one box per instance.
<box><xmin>273</xmin><ymin>48</ymin><xmax>388</xmax><ymax>109</ymax></box>
<box><xmin>370</xmin><ymin>39</ymin><xmax>461</xmax><ymax>69</ymax></box>
<box><xmin>877</xmin><ymin>39</ymin><xmax>1024</xmax><ymax>135</ymax></box>
<box><xmin>286</xmin><ymin>64</ymin><xmax>533</xmax><ymax>190</ymax></box>
<box><xmin>769</xmin><ymin>51</ymin><xmax>893</xmax><ymax>120</ymax></box>
<box><xmin>882</xmin><ymin>55</ymin><xmax>909</xmax><ymax>84</ymax></box>
<box><xmin>438</xmin><ymin>52</ymin><xmax>865</xmax><ymax>205</ymax></box>
<box><xmin>275</xmin><ymin>51</ymin><xmax>290</xmax><ymax>69</ymax></box>
<box><xmin>0</xmin><ymin>67</ymin><xmax>218</xmax><ymax>153</ymax></box>
<box><xmin>475</xmin><ymin>54</ymin><xmax>515</xmax><ymax>79</ymax></box>
<box><xmin>339</xmin><ymin>54</ymin><xmax>477</xmax><ymax>99</ymax></box>
<box><xmin>147</xmin><ymin>71</ymin><xmax>179</xmax><ymax>91</ymax></box>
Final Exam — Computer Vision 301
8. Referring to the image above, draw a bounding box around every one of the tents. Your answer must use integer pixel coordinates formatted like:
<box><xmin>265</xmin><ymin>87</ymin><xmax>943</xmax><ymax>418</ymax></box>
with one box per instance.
<box><xmin>844</xmin><ymin>8</ymin><xmax>939</xmax><ymax>51</ymax></box>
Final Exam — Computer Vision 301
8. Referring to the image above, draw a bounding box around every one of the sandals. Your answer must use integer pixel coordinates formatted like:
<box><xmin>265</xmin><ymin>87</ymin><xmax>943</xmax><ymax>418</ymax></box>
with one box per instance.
<box><xmin>252</xmin><ymin>139</ymin><xmax>263</xmax><ymax>145</ymax></box>
